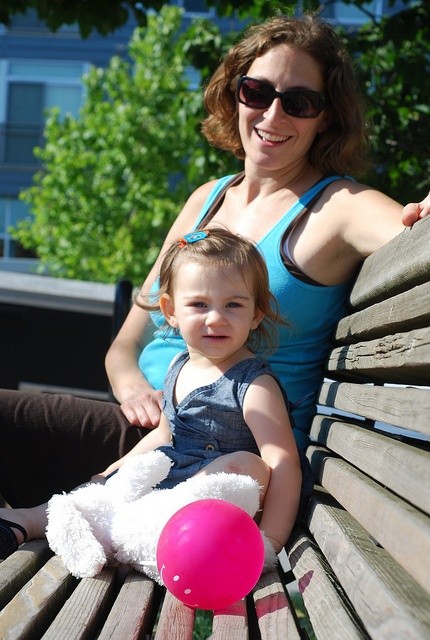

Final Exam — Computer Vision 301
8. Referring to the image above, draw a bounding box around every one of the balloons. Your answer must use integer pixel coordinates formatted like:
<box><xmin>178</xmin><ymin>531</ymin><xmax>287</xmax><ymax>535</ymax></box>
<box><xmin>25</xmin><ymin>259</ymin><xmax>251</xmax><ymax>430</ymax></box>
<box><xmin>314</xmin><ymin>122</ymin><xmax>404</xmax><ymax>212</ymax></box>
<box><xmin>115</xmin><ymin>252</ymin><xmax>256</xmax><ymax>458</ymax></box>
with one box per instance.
<box><xmin>157</xmin><ymin>499</ymin><xmax>264</xmax><ymax>610</ymax></box>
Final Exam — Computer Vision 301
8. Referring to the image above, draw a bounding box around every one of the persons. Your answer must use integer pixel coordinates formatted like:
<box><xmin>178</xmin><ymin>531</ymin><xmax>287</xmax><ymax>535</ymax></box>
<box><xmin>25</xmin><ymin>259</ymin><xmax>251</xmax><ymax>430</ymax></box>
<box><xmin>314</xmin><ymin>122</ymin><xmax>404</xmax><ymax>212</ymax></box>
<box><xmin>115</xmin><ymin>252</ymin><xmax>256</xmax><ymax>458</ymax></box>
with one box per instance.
<box><xmin>0</xmin><ymin>21</ymin><xmax>430</xmax><ymax>509</ymax></box>
<box><xmin>0</xmin><ymin>229</ymin><xmax>301</xmax><ymax>558</ymax></box>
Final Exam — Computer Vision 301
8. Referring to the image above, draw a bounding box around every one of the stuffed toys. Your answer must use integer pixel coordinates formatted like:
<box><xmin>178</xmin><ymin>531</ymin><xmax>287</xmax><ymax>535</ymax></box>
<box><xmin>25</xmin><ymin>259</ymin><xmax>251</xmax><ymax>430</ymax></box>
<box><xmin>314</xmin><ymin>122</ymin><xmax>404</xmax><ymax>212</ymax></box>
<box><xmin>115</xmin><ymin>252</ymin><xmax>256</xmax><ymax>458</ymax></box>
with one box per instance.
<box><xmin>45</xmin><ymin>452</ymin><xmax>279</xmax><ymax>590</ymax></box>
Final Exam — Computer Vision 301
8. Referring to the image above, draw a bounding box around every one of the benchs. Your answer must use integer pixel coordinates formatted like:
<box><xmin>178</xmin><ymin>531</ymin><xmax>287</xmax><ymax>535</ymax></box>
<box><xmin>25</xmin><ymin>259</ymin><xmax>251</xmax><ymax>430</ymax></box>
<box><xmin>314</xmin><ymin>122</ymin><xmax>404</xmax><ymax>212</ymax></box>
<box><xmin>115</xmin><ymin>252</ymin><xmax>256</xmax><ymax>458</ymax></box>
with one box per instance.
<box><xmin>1</xmin><ymin>212</ymin><xmax>430</xmax><ymax>640</ymax></box>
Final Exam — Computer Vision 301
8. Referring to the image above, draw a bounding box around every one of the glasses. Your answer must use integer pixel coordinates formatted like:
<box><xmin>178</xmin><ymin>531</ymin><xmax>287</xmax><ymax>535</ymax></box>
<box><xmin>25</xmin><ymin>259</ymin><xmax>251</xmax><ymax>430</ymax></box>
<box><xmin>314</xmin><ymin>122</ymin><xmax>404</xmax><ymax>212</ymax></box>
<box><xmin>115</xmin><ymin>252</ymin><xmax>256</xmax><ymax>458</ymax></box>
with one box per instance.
<box><xmin>237</xmin><ymin>75</ymin><xmax>330</xmax><ymax>118</ymax></box>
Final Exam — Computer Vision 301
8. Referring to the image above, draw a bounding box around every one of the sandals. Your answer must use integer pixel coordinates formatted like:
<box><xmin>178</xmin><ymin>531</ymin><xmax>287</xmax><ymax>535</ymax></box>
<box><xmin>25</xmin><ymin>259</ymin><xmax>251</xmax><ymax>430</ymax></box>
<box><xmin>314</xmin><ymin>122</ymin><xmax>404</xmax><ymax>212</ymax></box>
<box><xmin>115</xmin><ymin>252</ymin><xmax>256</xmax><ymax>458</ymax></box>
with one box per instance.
<box><xmin>0</xmin><ymin>518</ymin><xmax>28</xmax><ymax>560</ymax></box>
